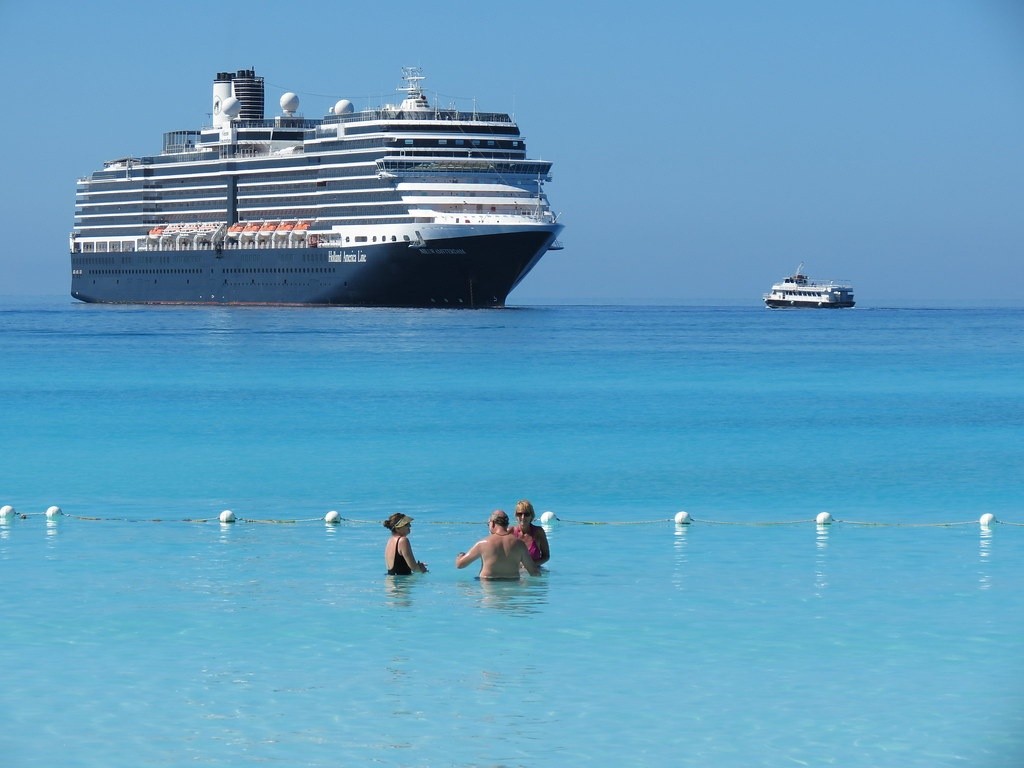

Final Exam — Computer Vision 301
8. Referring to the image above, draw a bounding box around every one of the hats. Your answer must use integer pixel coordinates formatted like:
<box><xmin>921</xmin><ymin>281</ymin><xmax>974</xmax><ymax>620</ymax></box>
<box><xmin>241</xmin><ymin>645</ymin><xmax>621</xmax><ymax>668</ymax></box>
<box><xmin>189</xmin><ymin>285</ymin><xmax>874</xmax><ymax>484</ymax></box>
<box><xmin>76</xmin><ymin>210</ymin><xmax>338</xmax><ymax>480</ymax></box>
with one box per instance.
<box><xmin>391</xmin><ymin>515</ymin><xmax>414</xmax><ymax>531</ymax></box>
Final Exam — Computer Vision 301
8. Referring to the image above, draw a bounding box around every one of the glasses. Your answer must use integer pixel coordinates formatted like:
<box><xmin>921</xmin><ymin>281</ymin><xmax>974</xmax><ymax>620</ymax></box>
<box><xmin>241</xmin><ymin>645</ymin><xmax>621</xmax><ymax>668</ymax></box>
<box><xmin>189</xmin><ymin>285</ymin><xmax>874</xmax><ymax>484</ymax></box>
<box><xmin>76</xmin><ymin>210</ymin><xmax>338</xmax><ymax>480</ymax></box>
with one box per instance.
<box><xmin>515</xmin><ymin>511</ymin><xmax>531</xmax><ymax>517</ymax></box>
<box><xmin>487</xmin><ymin>522</ymin><xmax>491</xmax><ymax>526</ymax></box>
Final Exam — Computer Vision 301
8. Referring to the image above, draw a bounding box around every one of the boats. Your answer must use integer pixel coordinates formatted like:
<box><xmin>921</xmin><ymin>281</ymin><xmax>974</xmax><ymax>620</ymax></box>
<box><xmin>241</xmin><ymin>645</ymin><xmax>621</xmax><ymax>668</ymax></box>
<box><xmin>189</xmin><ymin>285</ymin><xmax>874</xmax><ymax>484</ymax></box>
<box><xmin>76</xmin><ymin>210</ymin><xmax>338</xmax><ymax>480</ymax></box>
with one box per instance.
<box><xmin>762</xmin><ymin>274</ymin><xmax>862</xmax><ymax>308</ymax></box>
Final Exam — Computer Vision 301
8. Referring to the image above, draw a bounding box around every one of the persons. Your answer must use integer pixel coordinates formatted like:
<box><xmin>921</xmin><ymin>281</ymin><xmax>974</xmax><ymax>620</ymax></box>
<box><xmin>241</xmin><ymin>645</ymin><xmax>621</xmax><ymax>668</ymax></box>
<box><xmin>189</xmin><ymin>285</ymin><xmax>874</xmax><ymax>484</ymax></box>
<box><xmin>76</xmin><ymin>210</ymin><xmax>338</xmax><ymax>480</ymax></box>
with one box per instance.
<box><xmin>455</xmin><ymin>510</ymin><xmax>542</xmax><ymax>581</ymax></box>
<box><xmin>383</xmin><ymin>513</ymin><xmax>429</xmax><ymax>575</ymax></box>
<box><xmin>507</xmin><ymin>500</ymin><xmax>550</xmax><ymax>568</ymax></box>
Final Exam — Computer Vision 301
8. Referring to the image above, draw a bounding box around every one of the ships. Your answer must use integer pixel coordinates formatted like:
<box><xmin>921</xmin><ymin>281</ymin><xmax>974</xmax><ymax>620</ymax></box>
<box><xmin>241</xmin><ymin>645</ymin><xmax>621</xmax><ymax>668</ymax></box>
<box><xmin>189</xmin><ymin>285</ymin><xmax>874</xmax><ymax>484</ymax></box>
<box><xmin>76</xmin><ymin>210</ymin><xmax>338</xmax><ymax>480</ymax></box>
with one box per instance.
<box><xmin>68</xmin><ymin>66</ymin><xmax>566</xmax><ymax>308</ymax></box>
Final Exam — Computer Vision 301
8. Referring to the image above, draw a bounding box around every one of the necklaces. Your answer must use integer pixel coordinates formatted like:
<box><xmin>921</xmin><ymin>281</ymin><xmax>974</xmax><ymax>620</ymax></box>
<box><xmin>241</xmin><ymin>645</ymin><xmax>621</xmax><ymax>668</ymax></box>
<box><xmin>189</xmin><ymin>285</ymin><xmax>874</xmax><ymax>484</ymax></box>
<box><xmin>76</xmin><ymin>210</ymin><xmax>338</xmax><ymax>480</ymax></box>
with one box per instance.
<box><xmin>493</xmin><ymin>531</ymin><xmax>511</xmax><ymax>536</ymax></box>
